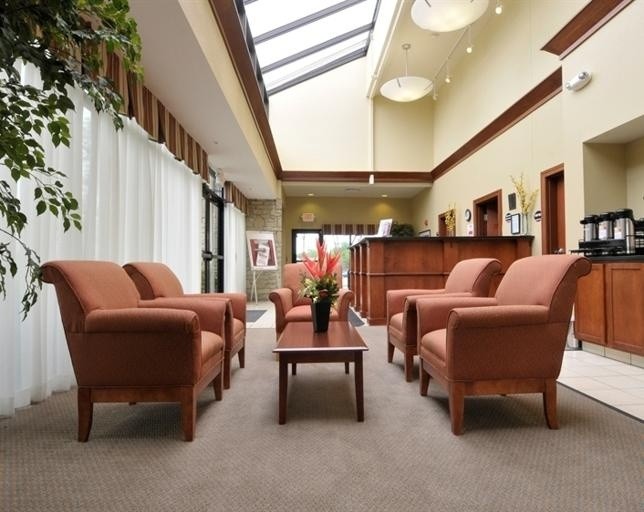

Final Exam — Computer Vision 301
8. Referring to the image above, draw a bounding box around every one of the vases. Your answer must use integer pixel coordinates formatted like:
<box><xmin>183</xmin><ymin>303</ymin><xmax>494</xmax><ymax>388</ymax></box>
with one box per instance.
<box><xmin>306</xmin><ymin>296</ymin><xmax>331</xmax><ymax>333</ymax></box>
<box><xmin>510</xmin><ymin>213</ymin><xmax>520</xmax><ymax>234</ymax></box>
<box><xmin>519</xmin><ymin>211</ymin><xmax>529</xmax><ymax>235</ymax></box>
<box><xmin>448</xmin><ymin>230</ymin><xmax>453</xmax><ymax>237</ymax></box>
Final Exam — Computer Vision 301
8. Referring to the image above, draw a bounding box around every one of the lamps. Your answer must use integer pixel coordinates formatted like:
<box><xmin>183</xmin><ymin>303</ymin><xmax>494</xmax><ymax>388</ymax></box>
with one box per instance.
<box><xmin>378</xmin><ymin>43</ymin><xmax>434</xmax><ymax>104</ymax></box>
<box><xmin>564</xmin><ymin>71</ymin><xmax>592</xmax><ymax>93</ymax></box>
<box><xmin>409</xmin><ymin>0</ymin><xmax>490</xmax><ymax>33</ymax></box>
<box><xmin>431</xmin><ymin>0</ymin><xmax>507</xmax><ymax>102</ymax></box>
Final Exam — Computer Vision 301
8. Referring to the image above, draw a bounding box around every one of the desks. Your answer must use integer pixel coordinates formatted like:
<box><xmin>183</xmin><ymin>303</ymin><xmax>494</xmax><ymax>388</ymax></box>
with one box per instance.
<box><xmin>273</xmin><ymin>321</ymin><xmax>370</xmax><ymax>423</ymax></box>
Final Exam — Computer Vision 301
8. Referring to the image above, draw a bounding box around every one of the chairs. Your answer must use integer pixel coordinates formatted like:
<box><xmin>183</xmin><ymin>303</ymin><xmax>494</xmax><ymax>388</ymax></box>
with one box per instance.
<box><xmin>120</xmin><ymin>260</ymin><xmax>249</xmax><ymax>390</ymax></box>
<box><xmin>413</xmin><ymin>254</ymin><xmax>595</xmax><ymax>435</ymax></box>
<box><xmin>36</xmin><ymin>260</ymin><xmax>230</xmax><ymax>443</ymax></box>
<box><xmin>268</xmin><ymin>259</ymin><xmax>354</xmax><ymax>333</ymax></box>
<box><xmin>384</xmin><ymin>257</ymin><xmax>503</xmax><ymax>385</ymax></box>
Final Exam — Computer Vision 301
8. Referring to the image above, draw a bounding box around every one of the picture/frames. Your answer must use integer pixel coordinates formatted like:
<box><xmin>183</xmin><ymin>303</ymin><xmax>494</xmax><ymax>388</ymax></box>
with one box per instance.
<box><xmin>417</xmin><ymin>229</ymin><xmax>431</xmax><ymax>238</ymax></box>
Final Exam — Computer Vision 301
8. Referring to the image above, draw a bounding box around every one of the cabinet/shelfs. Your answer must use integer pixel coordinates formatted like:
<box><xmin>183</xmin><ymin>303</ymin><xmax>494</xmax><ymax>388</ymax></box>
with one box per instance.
<box><xmin>572</xmin><ymin>257</ymin><xmax>644</xmax><ymax>354</ymax></box>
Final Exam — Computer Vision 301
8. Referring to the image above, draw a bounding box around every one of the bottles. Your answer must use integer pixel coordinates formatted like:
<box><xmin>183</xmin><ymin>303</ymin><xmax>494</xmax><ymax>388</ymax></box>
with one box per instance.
<box><xmin>580</xmin><ymin>214</ymin><xmax>600</xmax><ymax>257</ymax></box>
<box><xmin>613</xmin><ymin>208</ymin><xmax>637</xmax><ymax>256</ymax></box>
<box><xmin>598</xmin><ymin>212</ymin><xmax>615</xmax><ymax>257</ymax></box>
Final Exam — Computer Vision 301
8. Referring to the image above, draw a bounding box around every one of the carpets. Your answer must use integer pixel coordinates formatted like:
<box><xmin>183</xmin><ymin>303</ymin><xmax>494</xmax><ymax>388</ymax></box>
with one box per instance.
<box><xmin>0</xmin><ymin>324</ymin><xmax>643</xmax><ymax>511</ymax></box>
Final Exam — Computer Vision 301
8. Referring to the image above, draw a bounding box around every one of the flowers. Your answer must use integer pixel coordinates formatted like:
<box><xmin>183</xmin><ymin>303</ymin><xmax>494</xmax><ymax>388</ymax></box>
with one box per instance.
<box><xmin>294</xmin><ymin>238</ymin><xmax>343</xmax><ymax>310</ymax></box>
<box><xmin>510</xmin><ymin>170</ymin><xmax>538</xmax><ymax>215</ymax></box>
<box><xmin>444</xmin><ymin>203</ymin><xmax>456</xmax><ymax>231</ymax></box>
